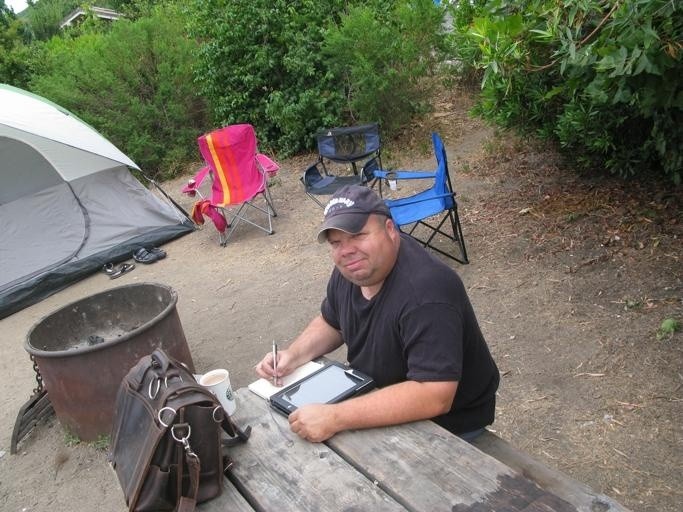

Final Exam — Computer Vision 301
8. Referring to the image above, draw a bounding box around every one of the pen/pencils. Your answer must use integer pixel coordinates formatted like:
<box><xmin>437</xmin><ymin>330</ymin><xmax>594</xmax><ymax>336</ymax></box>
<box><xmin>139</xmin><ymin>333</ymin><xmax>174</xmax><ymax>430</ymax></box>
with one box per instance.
<box><xmin>273</xmin><ymin>341</ymin><xmax>277</xmax><ymax>383</ymax></box>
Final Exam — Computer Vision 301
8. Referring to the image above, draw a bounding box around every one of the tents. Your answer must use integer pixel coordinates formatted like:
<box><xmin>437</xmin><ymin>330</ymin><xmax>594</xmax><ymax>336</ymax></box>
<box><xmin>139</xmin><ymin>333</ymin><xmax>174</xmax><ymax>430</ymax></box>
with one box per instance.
<box><xmin>0</xmin><ymin>82</ymin><xmax>195</xmax><ymax>319</ymax></box>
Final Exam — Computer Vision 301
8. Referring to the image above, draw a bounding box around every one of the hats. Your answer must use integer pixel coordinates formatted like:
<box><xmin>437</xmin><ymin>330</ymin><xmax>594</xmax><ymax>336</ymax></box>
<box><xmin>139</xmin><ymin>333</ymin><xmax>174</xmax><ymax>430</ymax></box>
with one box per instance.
<box><xmin>317</xmin><ymin>183</ymin><xmax>393</xmax><ymax>244</ymax></box>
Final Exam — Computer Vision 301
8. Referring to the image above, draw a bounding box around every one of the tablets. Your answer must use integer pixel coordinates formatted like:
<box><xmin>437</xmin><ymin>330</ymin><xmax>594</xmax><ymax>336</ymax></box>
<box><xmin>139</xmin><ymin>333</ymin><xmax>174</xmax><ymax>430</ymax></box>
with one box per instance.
<box><xmin>269</xmin><ymin>359</ymin><xmax>377</xmax><ymax>417</ymax></box>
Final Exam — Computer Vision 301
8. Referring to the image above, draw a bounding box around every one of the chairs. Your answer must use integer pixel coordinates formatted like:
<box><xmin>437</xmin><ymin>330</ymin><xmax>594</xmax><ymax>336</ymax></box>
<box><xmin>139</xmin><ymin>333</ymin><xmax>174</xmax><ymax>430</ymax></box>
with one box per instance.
<box><xmin>180</xmin><ymin>121</ymin><xmax>280</xmax><ymax>248</ymax></box>
<box><xmin>298</xmin><ymin>123</ymin><xmax>469</xmax><ymax>265</ymax></box>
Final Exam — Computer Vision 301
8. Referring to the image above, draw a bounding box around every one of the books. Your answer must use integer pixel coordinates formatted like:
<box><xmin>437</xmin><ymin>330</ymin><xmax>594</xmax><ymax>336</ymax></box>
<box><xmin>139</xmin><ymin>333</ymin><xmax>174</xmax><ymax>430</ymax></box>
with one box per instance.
<box><xmin>247</xmin><ymin>361</ymin><xmax>325</xmax><ymax>401</ymax></box>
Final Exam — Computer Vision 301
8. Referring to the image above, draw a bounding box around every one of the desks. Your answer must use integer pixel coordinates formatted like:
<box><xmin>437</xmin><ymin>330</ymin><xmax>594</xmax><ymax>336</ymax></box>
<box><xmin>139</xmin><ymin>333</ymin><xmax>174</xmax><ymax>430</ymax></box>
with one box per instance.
<box><xmin>182</xmin><ymin>355</ymin><xmax>575</xmax><ymax>512</ymax></box>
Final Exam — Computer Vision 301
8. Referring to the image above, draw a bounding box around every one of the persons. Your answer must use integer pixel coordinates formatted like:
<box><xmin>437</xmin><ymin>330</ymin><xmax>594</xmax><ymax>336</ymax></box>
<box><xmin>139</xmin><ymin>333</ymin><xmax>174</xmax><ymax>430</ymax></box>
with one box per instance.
<box><xmin>255</xmin><ymin>182</ymin><xmax>500</xmax><ymax>442</ymax></box>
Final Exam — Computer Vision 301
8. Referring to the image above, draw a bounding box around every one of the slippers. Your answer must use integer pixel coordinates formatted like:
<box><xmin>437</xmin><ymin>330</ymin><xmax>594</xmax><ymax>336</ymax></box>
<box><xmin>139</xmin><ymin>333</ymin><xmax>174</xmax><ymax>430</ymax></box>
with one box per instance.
<box><xmin>100</xmin><ymin>262</ymin><xmax>117</xmax><ymax>275</ymax></box>
<box><xmin>109</xmin><ymin>263</ymin><xmax>135</xmax><ymax>279</ymax></box>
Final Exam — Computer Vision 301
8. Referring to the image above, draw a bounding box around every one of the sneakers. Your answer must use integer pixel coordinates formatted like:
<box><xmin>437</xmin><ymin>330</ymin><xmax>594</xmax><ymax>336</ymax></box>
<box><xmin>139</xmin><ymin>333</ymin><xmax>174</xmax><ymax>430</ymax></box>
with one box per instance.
<box><xmin>132</xmin><ymin>246</ymin><xmax>158</xmax><ymax>264</ymax></box>
<box><xmin>146</xmin><ymin>246</ymin><xmax>166</xmax><ymax>260</ymax></box>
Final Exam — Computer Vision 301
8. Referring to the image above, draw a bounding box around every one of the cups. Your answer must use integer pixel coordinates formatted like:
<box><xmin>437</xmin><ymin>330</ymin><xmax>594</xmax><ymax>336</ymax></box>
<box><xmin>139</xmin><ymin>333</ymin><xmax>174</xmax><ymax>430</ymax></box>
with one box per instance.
<box><xmin>201</xmin><ymin>368</ymin><xmax>238</xmax><ymax>418</ymax></box>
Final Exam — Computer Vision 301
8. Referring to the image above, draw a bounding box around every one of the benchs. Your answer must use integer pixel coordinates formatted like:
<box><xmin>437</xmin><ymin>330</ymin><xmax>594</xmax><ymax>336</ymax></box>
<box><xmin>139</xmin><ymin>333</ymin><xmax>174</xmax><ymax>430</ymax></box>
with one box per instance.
<box><xmin>465</xmin><ymin>429</ymin><xmax>632</xmax><ymax>512</ymax></box>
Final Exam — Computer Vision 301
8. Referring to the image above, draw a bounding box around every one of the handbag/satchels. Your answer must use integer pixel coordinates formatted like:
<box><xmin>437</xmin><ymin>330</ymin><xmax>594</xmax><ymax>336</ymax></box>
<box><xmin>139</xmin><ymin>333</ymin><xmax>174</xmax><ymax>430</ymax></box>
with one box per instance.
<box><xmin>107</xmin><ymin>346</ymin><xmax>252</xmax><ymax>512</ymax></box>
